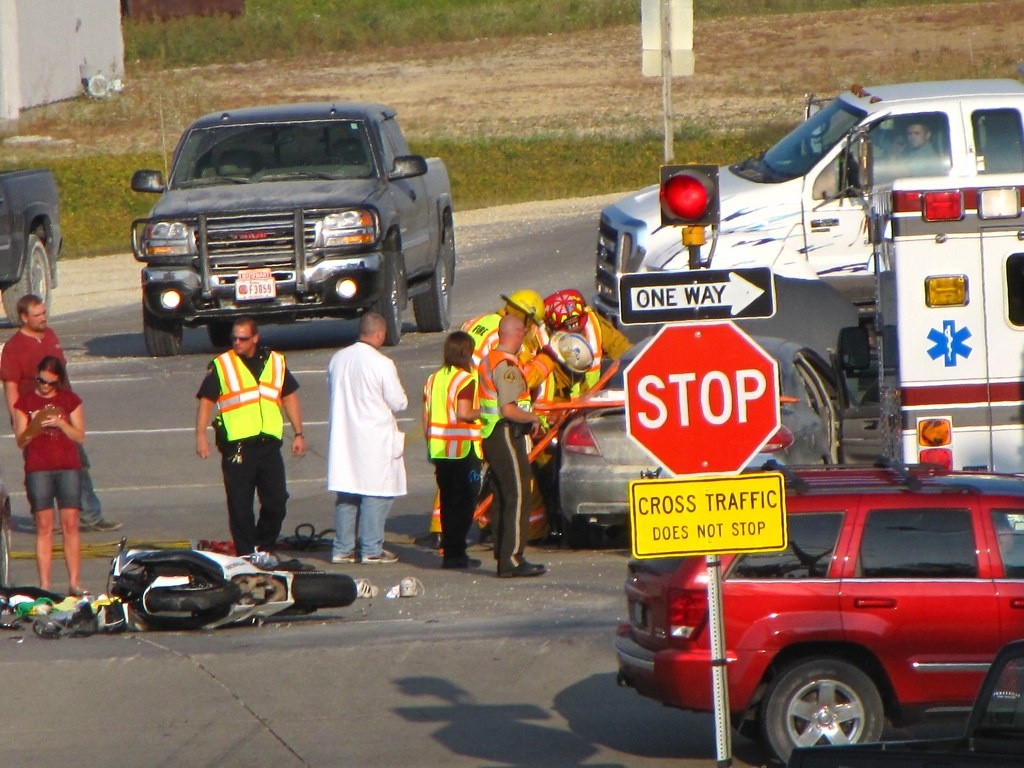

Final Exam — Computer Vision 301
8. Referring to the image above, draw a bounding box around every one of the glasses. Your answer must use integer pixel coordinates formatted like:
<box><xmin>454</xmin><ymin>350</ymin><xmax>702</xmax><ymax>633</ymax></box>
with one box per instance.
<box><xmin>36</xmin><ymin>377</ymin><xmax>58</xmax><ymax>387</ymax></box>
<box><xmin>229</xmin><ymin>335</ymin><xmax>254</xmax><ymax>341</ymax></box>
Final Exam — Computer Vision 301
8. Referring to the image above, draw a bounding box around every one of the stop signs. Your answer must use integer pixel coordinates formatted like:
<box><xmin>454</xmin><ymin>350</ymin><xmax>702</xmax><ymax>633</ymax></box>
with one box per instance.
<box><xmin>624</xmin><ymin>320</ymin><xmax>781</xmax><ymax>479</ymax></box>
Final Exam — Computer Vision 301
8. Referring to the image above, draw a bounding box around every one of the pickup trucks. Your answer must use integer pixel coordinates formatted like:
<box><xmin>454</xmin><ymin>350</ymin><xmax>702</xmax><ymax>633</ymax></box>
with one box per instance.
<box><xmin>0</xmin><ymin>167</ymin><xmax>66</xmax><ymax>329</ymax></box>
<box><xmin>788</xmin><ymin>638</ymin><xmax>1024</xmax><ymax>768</ymax></box>
<box><xmin>131</xmin><ymin>102</ymin><xmax>457</xmax><ymax>358</ymax></box>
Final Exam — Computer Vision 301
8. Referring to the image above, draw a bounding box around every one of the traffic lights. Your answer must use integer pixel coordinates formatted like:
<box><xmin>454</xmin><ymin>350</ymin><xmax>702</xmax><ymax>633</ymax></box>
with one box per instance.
<box><xmin>659</xmin><ymin>164</ymin><xmax>720</xmax><ymax>226</ymax></box>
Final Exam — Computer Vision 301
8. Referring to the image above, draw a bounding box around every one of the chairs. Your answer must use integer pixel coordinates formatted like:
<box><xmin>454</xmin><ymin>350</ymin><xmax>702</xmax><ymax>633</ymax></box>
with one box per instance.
<box><xmin>331</xmin><ymin>138</ymin><xmax>366</xmax><ymax>164</ymax></box>
<box><xmin>930</xmin><ymin>130</ymin><xmax>943</xmax><ymax>161</ymax></box>
<box><xmin>218</xmin><ymin>149</ymin><xmax>254</xmax><ymax>178</ymax></box>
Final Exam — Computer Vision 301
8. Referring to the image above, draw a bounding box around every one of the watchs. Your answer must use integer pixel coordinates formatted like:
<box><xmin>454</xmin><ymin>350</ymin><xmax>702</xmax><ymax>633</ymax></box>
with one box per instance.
<box><xmin>295</xmin><ymin>432</ymin><xmax>304</xmax><ymax>438</ymax></box>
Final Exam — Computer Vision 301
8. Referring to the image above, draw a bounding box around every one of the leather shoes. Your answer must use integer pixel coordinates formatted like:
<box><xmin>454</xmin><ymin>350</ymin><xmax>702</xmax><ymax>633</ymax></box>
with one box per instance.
<box><xmin>498</xmin><ymin>561</ymin><xmax>546</xmax><ymax>578</ymax></box>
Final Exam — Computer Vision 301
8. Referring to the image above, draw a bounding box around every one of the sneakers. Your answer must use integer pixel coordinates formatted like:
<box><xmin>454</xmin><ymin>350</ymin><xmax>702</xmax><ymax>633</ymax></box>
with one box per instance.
<box><xmin>331</xmin><ymin>553</ymin><xmax>361</xmax><ymax>563</ymax></box>
<box><xmin>80</xmin><ymin>517</ymin><xmax>123</xmax><ymax>531</ymax></box>
<box><xmin>361</xmin><ymin>550</ymin><xmax>400</xmax><ymax>564</ymax></box>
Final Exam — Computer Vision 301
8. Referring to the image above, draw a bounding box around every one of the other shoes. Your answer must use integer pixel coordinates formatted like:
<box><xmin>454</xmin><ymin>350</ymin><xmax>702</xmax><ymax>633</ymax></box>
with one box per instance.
<box><xmin>440</xmin><ymin>559</ymin><xmax>481</xmax><ymax>570</ymax></box>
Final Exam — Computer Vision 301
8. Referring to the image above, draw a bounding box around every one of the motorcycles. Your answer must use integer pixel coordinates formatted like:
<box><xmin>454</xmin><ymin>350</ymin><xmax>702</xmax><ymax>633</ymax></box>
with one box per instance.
<box><xmin>107</xmin><ymin>537</ymin><xmax>358</xmax><ymax>631</ymax></box>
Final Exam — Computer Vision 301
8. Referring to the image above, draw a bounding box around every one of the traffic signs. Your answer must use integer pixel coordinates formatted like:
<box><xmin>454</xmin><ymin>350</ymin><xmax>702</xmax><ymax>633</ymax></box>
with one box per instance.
<box><xmin>617</xmin><ymin>266</ymin><xmax>778</xmax><ymax>326</ymax></box>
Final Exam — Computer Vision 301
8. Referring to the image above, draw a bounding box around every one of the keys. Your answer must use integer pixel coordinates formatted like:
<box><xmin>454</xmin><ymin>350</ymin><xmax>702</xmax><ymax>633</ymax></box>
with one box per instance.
<box><xmin>228</xmin><ymin>444</ymin><xmax>242</xmax><ymax>463</ymax></box>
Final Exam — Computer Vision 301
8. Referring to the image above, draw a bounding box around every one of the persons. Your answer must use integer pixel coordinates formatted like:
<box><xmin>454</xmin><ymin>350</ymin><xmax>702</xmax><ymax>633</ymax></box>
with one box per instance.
<box><xmin>0</xmin><ymin>294</ymin><xmax>123</xmax><ymax>531</ymax></box>
<box><xmin>13</xmin><ymin>355</ymin><xmax>89</xmax><ymax>597</ymax></box>
<box><xmin>196</xmin><ymin>317</ymin><xmax>304</xmax><ymax>556</ymax></box>
<box><xmin>887</xmin><ymin>121</ymin><xmax>938</xmax><ymax>177</ymax></box>
<box><xmin>421</xmin><ymin>288</ymin><xmax>634</xmax><ymax>578</ymax></box>
<box><xmin>326</xmin><ymin>312</ymin><xmax>408</xmax><ymax>563</ymax></box>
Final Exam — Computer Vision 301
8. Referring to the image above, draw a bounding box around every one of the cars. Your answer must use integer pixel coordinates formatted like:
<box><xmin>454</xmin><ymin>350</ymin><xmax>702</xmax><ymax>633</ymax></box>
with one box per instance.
<box><xmin>557</xmin><ymin>330</ymin><xmax>849</xmax><ymax>549</ymax></box>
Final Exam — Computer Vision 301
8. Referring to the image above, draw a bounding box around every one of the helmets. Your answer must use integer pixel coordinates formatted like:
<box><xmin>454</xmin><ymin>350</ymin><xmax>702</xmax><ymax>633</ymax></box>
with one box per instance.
<box><xmin>500</xmin><ymin>289</ymin><xmax>545</xmax><ymax>327</ymax></box>
<box><xmin>544</xmin><ymin>289</ymin><xmax>588</xmax><ymax>333</ymax></box>
<box><xmin>550</xmin><ymin>331</ymin><xmax>594</xmax><ymax>373</ymax></box>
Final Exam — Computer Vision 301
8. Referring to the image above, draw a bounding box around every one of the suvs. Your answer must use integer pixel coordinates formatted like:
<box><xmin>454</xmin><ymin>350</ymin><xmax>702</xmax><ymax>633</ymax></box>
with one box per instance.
<box><xmin>591</xmin><ymin>78</ymin><xmax>1024</xmax><ymax>346</ymax></box>
<box><xmin>614</xmin><ymin>456</ymin><xmax>1024</xmax><ymax>768</ymax></box>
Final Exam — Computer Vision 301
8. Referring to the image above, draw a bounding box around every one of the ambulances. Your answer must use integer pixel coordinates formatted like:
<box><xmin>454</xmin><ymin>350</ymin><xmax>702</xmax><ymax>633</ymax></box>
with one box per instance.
<box><xmin>866</xmin><ymin>174</ymin><xmax>1024</xmax><ymax>480</ymax></box>
